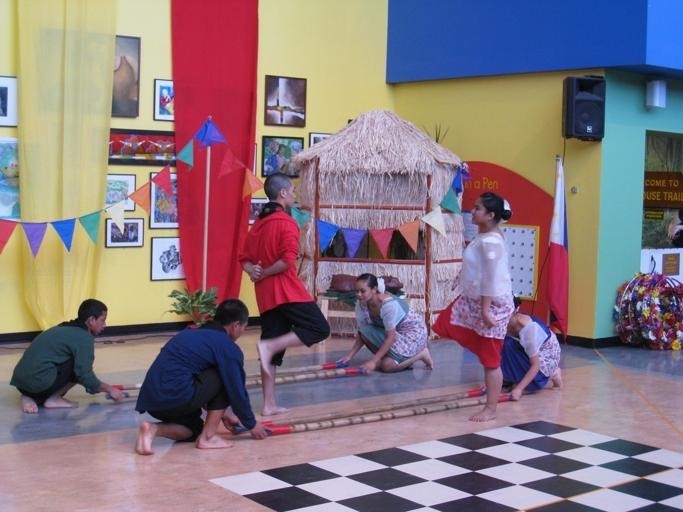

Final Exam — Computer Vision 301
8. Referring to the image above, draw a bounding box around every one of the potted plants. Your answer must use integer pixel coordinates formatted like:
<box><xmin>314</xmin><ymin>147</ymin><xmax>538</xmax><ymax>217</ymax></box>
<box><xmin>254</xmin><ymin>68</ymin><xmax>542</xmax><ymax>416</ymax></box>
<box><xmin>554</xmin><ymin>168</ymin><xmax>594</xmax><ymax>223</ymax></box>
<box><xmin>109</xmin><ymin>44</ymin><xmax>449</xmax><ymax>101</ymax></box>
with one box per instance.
<box><xmin>161</xmin><ymin>286</ymin><xmax>223</xmax><ymax>332</ymax></box>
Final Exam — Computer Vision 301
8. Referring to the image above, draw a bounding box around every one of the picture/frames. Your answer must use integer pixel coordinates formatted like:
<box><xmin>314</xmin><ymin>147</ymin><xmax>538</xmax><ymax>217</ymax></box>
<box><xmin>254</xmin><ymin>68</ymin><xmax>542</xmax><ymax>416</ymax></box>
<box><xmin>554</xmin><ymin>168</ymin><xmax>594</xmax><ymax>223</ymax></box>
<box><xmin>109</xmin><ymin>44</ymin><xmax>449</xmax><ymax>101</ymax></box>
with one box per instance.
<box><xmin>103</xmin><ymin>172</ymin><xmax>137</xmax><ymax>213</ymax></box>
<box><xmin>108</xmin><ymin>127</ymin><xmax>177</xmax><ymax>167</ymax></box>
<box><xmin>0</xmin><ymin>74</ymin><xmax>19</xmax><ymax>130</ymax></box>
<box><xmin>247</xmin><ymin>73</ymin><xmax>334</xmax><ymax>226</ymax></box>
<box><xmin>110</xmin><ymin>34</ymin><xmax>142</xmax><ymax>119</ymax></box>
<box><xmin>104</xmin><ymin>216</ymin><xmax>145</xmax><ymax>249</ymax></box>
<box><xmin>149</xmin><ymin>236</ymin><xmax>186</xmax><ymax>282</ymax></box>
<box><xmin>148</xmin><ymin>170</ymin><xmax>178</xmax><ymax>230</ymax></box>
<box><xmin>152</xmin><ymin>77</ymin><xmax>174</xmax><ymax>124</ymax></box>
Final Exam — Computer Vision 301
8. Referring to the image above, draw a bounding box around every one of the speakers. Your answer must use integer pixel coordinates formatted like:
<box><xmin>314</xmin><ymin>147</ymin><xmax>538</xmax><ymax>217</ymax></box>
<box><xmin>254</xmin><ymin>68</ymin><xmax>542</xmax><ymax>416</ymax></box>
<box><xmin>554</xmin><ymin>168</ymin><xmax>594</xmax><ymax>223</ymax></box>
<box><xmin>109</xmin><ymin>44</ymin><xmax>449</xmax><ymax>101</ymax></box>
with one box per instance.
<box><xmin>562</xmin><ymin>75</ymin><xmax>606</xmax><ymax>141</ymax></box>
<box><xmin>646</xmin><ymin>80</ymin><xmax>666</xmax><ymax>109</ymax></box>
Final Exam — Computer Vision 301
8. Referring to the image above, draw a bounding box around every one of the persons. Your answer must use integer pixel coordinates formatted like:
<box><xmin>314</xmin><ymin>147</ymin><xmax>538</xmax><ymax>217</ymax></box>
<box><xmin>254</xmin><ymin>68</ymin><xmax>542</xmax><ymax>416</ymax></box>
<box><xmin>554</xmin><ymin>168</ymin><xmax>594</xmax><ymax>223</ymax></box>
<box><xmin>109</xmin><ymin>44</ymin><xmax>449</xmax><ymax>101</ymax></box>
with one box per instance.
<box><xmin>160</xmin><ymin>89</ymin><xmax>172</xmax><ymax>107</ymax></box>
<box><xmin>333</xmin><ymin>272</ymin><xmax>435</xmax><ymax>375</ymax></box>
<box><xmin>477</xmin><ymin>291</ymin><xmax>564</xmax><ymax>402</ymax></box>
<box><xmin>237</xmin><ymin>172</ymin><xmax>332</xmax><ymax>417</ymax></box>
<box><xmin>7</xmin><ymin>298</ymin><xmax>126</xmax><ymax>415</ymax></box>
<box><xmin>128</xmin><ymin>225</ymin><xmax>138</xmax><ymax>243</ymax></box>
<box><xmin>667</xmin><ymin>206</ymin><xmax>683</xmax><ymax>247</ymax></box>
<box><xmin>264</xmin><ymin>140</ymin><xmax>302</xmax><ymax>175</ymax></box>
<box><xmin>430</xmin><ymin>191</ymin><xmax>515</xmax><ymax>423</ymax></box>
<box><xmin>134</xmin><ymin>298</ymin><xmax>277</xmax><ymax>456</ymax></box>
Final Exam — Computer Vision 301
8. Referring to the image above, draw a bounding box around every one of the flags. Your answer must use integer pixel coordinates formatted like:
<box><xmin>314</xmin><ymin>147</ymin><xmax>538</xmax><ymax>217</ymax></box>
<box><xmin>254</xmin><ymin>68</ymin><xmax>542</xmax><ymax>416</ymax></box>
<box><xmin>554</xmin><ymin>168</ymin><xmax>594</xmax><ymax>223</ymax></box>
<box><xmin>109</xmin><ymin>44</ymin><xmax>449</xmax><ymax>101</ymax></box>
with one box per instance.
<box><xmin>543</xmin><ymin>155</ymin><xmax>571</xmax><ymax>344</ymax></box>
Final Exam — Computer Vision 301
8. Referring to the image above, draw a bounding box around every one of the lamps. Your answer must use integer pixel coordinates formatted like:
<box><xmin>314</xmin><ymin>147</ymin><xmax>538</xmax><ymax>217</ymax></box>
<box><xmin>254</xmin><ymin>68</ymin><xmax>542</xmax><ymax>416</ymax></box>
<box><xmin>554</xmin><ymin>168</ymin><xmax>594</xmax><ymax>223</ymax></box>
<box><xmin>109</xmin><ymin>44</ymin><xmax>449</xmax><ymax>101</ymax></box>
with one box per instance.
<box><xmin>645</xmin><ymin>77</ymin><xmax>666</xmax><ymax>112</ymax></box>
